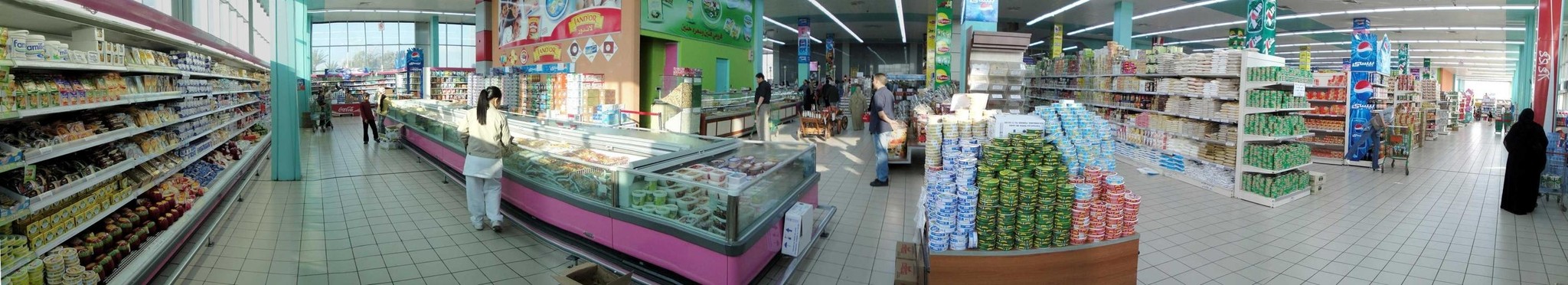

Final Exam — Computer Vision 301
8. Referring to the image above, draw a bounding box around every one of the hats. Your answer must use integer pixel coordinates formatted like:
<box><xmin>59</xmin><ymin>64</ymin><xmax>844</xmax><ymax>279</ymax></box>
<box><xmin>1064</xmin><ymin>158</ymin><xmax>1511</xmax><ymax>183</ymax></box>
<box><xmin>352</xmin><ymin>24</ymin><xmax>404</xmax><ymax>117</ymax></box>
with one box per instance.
<box><xmin>378</xmin><ymin>88</ymin><xmax>385</xmax><ymax>94</ymax></box>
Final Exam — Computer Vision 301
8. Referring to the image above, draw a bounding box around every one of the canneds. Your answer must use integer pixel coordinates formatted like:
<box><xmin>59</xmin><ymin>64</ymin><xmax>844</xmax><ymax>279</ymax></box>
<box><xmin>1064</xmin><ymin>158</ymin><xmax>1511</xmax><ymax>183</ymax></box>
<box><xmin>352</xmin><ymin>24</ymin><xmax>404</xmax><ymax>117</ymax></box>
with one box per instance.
<box><xmin>1241</xmin><ymin>66</ymin><xmax>1311</xmax><ymax>198</ymax></box>
<box><xmin>1367</xmin><ymin>73</ymin><xmax>1389</xmax><ymax>98</ymax></box>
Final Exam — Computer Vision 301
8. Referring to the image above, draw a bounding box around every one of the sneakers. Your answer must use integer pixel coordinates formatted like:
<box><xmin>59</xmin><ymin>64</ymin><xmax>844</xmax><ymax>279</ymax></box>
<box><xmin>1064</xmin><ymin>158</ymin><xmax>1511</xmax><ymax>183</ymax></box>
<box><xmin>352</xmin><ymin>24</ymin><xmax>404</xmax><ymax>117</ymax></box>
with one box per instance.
<box><xmin>472</xmin><ymin>223</ymin><xmax>483</xmax><ymax>230</ymax></box>
<box><xmin>374</xmin><ymin>139</ymin><xmax>379</xmax><ymax>143</ymax></box>
<box><xmin>364</xmin><ymin>141</ymin><xmax>368</xmax><ymax>143</ymax></box>
<box><xmin>493</xmin><ymin>219</ymin><xmax>502</xmax><ymax>231</ymax></box>
<box><xmin>870</xmin><ymin>179</ymin><xmax>889</xmax><ymax>186</ymax></box>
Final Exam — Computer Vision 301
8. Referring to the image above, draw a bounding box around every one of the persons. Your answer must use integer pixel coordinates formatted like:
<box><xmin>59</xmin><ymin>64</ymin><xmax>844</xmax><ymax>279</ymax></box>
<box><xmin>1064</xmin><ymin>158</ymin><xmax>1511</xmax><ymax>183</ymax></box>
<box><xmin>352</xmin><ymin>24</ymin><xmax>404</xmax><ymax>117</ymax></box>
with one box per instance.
<box><xmin>300</xmin><ymin>83</ymin><xmax>304</xmax><ymax>90</ymax></box>
<box><xmin>869</xmin><ymin>73</ymin><xmax>899</xmax><ymax>186</ymax></box>
<box><xmin>318</xmin><ymin>80</ymin><xmax>366</xmax><ymax>128</ymax></box>
<box><xmin>886</xmin><ymin>77</ymin><xmax>908</xmax><ymax>101</ymax></box>
<box><xmin>360</xmin><ymin>92</ymin><xmax>381</xmax><ymax>144</ymax></box>
<box><xmin>1488</xmin><ymin>110</ymin><xmax>1493</xmax><ymax>126</ymax></box>
<box><xmin>1501</xmin><ymin>105</ymin><xmax>1505</xmax><ymax>122</ymax></box>
<box><xmin>1510</xmin><ymin>103</ymin><xmax>1514</xmax><ymax>120</ymax></box>
<box><xmin>458</xmin><ymin>85</ymin><xmax>513</xmax><ymax>232</ymax></box>
<box><xmin>1345</xmin><ymin>104</ymin><xmax>1406</xmax><ymax>171</ymax></box>
<box><xmin>1500</xmin><ymin>106</ymin><xmax>1549</xmax><ymax>215</ymax></box>
<box><xmin>376</xmin><ymin>95</ymin><xmax>392</xmax><ymax>134</ymax></box>
<box><xmin>1474</xmin><ymin>111</ymin><xmax>1481</xmax><ymax>121</ymax></box>
<box><xmin>849</xmin><ymin>85</ymin><xmax>868</xmax><ymax>131</ymax></box>
<box><xmin>754</xmin><ymin>73</ymin><xmax>771</xmax><ymax>142</ymax></box>
<box><xmin>798</xmin><ymin>73</ymin><xmax>851</xmax><ymax>109</ymax></box>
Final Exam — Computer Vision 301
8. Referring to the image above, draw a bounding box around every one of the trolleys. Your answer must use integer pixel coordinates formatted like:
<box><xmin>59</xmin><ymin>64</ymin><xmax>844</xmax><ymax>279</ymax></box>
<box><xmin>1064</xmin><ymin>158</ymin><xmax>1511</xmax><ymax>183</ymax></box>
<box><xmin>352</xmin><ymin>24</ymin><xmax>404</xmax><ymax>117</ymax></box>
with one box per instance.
<box><xmin>748</xmin><ymin>101</ymin><xmax>779</xmax><ymax>139</ymax></box>
<box><xmin>1535</xmin><ymin>147</ymin><xmax>1567</xmax><ymax>212</ymax></box>
<box><xmin>1381</xmin><ymin>124</ymin><xmax>1412</xmax><ymax>175</ymax></box>
<box><xmin>310</xmin><ymin>98</ymin><xmax>334</xmax><ymax>132</ymax></box>
<box><xmin>798</xmin><ymin>110</ymin><xmax>848</xmax><ymax>141</ymax></box>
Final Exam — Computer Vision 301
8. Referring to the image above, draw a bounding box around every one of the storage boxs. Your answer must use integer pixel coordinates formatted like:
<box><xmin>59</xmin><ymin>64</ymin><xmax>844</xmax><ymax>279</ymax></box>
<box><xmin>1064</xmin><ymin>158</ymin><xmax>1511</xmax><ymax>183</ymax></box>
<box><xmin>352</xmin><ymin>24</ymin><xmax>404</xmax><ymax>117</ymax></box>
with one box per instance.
<box><xmin>781</xmin><ymin>201</ymin><xmax>814</xmax><ymax>258</ymax></box>
<box><xmin>378</xmin><ymin>132</ymin><xmax>400</xmax><ymax>151</ymax></box>
<box><xmin>385</xmin><ymin>123</ymin><xmax>405</xmax><ymax>140</ymax></box>
<box><xmin>896</xmin><ymin>241</ymin><xmax>917</xmax><ymax>260</ymax></box>
<box><xmin>551</xmin><ymin>73</ymin><xmax>630</xmax><ymax>124</ymax></box>
<box><xmin>894</xmin><ymin>258</ymin><xmax>918</xmax><ymax>285</ymax></box>
<box><xmin>551</xmin><ymin>262</ymin><xmax>634</xmax><ymax>285</ymax></box>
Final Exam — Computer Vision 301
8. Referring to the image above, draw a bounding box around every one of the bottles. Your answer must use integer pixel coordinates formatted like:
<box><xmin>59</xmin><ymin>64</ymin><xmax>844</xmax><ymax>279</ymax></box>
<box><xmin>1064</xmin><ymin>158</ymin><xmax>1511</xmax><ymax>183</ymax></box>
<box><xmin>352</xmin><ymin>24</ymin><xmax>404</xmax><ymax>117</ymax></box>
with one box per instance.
<box><xmin>1105</xmin><ymin>94</ymin><xmax>1152</xmax><ymax>124</ymax></box>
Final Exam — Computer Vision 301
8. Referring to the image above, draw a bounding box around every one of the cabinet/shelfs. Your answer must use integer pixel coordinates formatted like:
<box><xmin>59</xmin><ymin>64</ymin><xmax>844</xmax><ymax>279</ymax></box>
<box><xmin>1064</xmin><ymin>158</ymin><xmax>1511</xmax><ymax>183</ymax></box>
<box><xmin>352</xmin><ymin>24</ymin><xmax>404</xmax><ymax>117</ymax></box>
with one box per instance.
<box><xmin>311</xmin><ymin>67</ymin><xmax>475</xmax><ymax>107</ymax></box>
<box><xmin>0</xmin><ymin>0</ymin><xmax>271</xmax><ymax>285</ymax></box>
